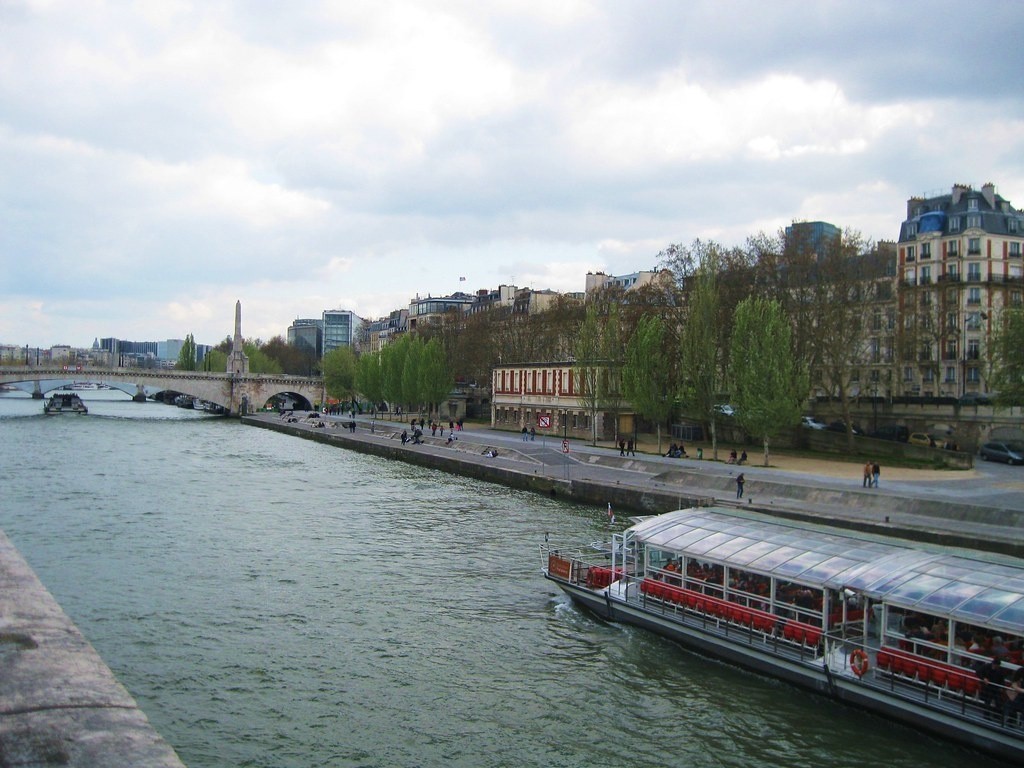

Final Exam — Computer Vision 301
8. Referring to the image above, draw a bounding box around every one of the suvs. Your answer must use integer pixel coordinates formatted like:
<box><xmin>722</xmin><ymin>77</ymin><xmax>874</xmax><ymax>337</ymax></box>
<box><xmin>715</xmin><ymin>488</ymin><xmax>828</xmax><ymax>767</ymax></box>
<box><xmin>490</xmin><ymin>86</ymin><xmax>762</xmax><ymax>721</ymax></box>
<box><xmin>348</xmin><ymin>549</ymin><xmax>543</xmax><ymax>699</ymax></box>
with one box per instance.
<box><xmin>980</xmin><ymin>441</ymin><xmax>1024</xmax><ymax>465</ymax></box>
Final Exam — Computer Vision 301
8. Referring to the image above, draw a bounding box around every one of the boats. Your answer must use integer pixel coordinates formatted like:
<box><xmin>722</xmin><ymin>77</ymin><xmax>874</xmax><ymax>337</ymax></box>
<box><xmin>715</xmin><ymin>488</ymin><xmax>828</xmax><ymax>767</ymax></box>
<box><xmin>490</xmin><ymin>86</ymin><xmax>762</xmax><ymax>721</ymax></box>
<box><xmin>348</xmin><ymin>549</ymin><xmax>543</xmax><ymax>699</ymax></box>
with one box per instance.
<box><xmin>43</xmin><ymin>393</ymin><xmax>88</xmax><ymax>416</ymax></box>
<box><xmin>538</xmin><ymin>505</ymin><xmax>1024</xmax><ymax>768</ymax></box>
<box><xmin>174</xmin><ymin>395</ymin><xmax>224</xmax><ymax>413</ymax></box>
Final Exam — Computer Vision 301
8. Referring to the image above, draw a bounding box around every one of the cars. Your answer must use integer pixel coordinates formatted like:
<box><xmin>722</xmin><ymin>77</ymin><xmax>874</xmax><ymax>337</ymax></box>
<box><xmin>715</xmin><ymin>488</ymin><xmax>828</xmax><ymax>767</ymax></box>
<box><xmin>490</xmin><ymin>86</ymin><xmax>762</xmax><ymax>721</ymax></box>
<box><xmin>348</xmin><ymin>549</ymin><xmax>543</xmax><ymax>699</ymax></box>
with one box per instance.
<box><xmin>957</xmin><ymin>391</ymin><xmax>989</xmax><ymax>405</ymax></box>
<box><xmin>709</xmin><ymin>403</ymin><xmax>950</xmax><ymax>449</ymax></box>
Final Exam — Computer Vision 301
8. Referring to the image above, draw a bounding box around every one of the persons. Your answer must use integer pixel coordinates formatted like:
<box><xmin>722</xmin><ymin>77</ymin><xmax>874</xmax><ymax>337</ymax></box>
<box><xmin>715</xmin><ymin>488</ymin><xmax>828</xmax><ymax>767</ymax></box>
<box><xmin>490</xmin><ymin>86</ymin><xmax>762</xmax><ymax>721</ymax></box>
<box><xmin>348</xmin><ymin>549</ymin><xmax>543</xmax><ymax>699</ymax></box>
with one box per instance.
<box><xmin>522</xmin><ymin>426</ymin><xmax>528</xmax><ymax>441</ymax></box>
<box><xmin>280</xmin><ymin>402</ymin><xmax>498</xmax><ymax>459</ymax></box>
<box><xmin>863</xmin><ymin>460</ymin><xmax>872</xmax><ymax>488</ymax></box>
<box><xmin>736</xmin><ymin>473</ymin><xmax>745</xmax><ymax>499</ymax></box>
<box><xmin>619</xmin><ymin>438</ymin><xmax>748</xmax><ymax>465</ymax></box>
<box><xmin>662</xmin><ymin>555</ymin><xmax>1024</xmax><ymax>730</ymax></box>
<box><xmin>530</xmin><ymin>425</ymin><xmax>535</xmax><ymax>441</ymax></box>
<box><xmin>871</xmin><ymin>461</ymin><xmax>881</xmax><ymax>490</ymax></box>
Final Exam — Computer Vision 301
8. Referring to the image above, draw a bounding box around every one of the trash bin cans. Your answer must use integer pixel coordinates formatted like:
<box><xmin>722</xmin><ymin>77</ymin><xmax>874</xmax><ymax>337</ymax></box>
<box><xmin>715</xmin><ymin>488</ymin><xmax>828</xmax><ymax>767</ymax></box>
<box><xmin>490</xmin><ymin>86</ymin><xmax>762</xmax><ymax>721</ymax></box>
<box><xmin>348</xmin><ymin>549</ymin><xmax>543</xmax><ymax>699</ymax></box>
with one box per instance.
<box><xmin>697</xmin><ymin>448</ymin><xmax>703</xmax><ymax>459</ymax></box>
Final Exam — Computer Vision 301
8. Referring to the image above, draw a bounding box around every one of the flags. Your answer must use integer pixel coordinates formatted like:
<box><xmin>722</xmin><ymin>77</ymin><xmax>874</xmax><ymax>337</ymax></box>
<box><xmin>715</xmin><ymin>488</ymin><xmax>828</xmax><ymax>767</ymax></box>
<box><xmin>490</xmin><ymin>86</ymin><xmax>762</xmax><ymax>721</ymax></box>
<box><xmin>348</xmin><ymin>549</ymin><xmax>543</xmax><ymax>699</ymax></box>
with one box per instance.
<box><xmin>608</xmin><ymin>505</ymin><xmax>616</xmax><ymax>524</ymax></box>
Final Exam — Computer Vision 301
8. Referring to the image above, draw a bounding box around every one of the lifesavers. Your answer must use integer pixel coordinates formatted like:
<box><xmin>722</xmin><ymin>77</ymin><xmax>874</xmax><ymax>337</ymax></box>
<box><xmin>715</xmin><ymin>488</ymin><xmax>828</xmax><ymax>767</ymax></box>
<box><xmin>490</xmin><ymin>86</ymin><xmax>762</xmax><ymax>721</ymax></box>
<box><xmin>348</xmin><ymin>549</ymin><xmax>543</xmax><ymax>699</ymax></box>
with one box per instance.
<box><xmin>850</xmin><ymin>649</ymin><xmax>868</xmax><ymax>675</ymax></box>
<box><xmin>587</xmin><ymin>567</ymin><xmax>628</xmax><ymax>588</ymax></box>
